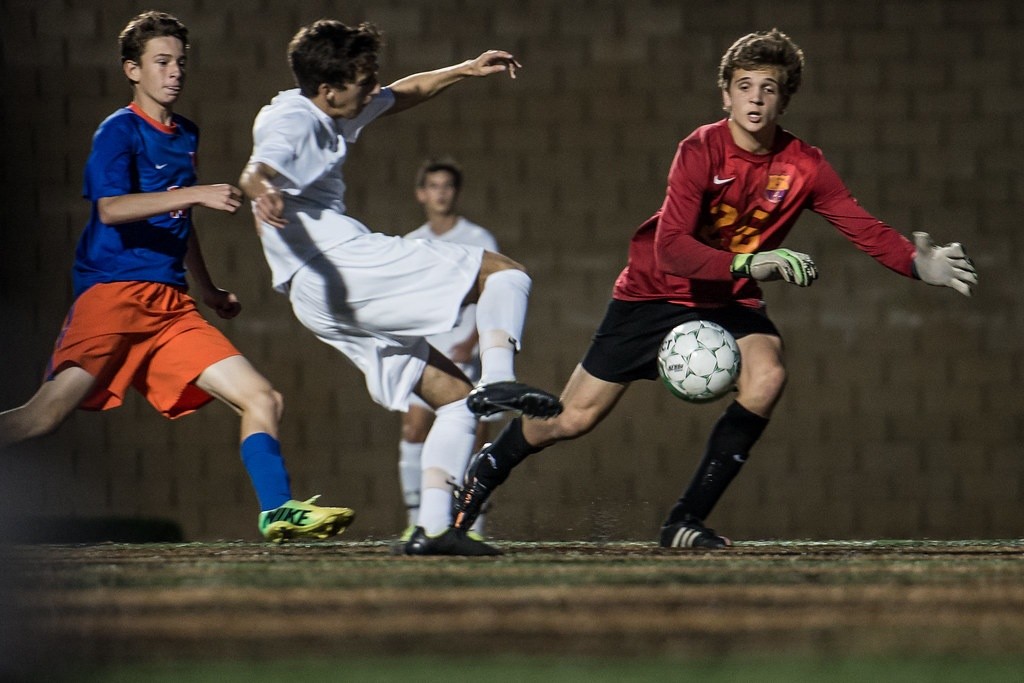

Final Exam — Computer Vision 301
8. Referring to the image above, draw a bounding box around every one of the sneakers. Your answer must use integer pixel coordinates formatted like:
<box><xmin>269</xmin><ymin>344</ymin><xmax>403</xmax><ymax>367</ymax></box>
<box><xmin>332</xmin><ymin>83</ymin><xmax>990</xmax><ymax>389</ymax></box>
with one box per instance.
<box><xmin>450</xmin><ymin>441</ymin><xmax>495</xmax><ymax>531</ymax></box>
<box><xmin>658</xmin><ymin>502</ymin><xmax>733</xmax><ymax>550</ymax></box>
<box><xmin>400</xmin><ymin>522</ymin><xmax>486</xmax><ymax>543</ymax></box>
<box><xmin>466</xmin><ymin>374</ymin><xmax>565</xmax><ymax>421</ymax></box>
<box><xmin>257</xmin><ymin>493</ymin><xmax>354</xmax><ymax>543</ymax></box>
<box><xmin>405</xmin><ymin>525</ymin><xmax>504</xmax><ymax>557</ymax></box>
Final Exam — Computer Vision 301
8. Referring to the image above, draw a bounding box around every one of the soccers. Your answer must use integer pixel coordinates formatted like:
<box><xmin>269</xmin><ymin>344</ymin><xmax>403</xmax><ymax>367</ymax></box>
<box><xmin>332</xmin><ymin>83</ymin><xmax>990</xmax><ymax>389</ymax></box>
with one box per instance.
<box><xmin>656</xmin><ymin>320</ymin><xmax>745</xmax><ymax>406</ymax></box>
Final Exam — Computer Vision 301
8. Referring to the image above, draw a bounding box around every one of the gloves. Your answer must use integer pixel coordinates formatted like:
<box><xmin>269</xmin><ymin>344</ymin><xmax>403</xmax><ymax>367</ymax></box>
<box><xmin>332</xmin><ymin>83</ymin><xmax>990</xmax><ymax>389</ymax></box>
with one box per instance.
<box><xmin>729</xmin><ymin>248</ymin><xmax>817</xmax><ymax>287</ymax></box>
<box><xmin>911</xmin><ymin>230</ymin><xmax>978</xmax><ymax>300</ymax></box>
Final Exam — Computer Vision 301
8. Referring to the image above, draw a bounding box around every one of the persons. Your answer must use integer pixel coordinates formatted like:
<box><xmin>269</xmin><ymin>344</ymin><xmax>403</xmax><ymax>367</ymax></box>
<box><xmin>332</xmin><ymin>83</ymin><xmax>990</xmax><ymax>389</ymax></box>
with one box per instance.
<box><xmin>0</xmin><ymin>7</ymin><xmax>355</xmax><ymax>548</ymax></box>
<box><xmin>396</xmin><ymin>155</ymin><xmax>492</xmax><ymax>546</ymax></box>
<box><xmin>451</xmin><ymin>28</ymin><xmax>976</xmax><ymax>551</ymax></box>
<box><xmin>238</xmin><ymin>16</ymin><xmax>567</xmax><ymax>558</ymax></box>
